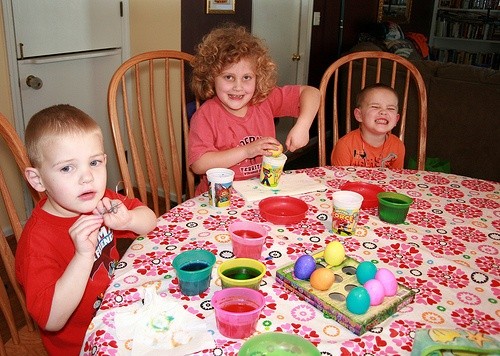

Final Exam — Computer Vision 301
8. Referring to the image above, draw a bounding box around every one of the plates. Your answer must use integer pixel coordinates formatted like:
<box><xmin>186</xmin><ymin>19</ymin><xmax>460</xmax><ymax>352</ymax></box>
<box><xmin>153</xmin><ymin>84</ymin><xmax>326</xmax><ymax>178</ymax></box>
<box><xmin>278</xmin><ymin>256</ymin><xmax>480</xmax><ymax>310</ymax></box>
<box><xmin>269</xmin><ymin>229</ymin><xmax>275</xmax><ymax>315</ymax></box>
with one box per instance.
<box><xmin>259</xmin><ymin>196</ymin><xmax>309</xmax><ymax>224</ymax></box>
<box><xmin>340</xmin><ymin>182</ymin><xmax>386</xmax><ymax>210</ymax></box>
<box><xmin>238</xmin><ymin>332</ymin><xmax>321</xmax><ymax>356</ymax></box>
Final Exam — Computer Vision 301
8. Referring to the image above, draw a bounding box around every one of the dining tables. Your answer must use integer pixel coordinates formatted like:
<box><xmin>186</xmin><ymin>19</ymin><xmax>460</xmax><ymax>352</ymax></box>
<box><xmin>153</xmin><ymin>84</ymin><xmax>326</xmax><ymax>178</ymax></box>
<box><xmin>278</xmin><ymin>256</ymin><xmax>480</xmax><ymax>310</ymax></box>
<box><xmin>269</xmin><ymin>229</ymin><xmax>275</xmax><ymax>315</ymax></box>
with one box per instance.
<box><xmin>74</xmin><ymin>164</ymin><xmax>500</xmax><ymax>356</ymax></box>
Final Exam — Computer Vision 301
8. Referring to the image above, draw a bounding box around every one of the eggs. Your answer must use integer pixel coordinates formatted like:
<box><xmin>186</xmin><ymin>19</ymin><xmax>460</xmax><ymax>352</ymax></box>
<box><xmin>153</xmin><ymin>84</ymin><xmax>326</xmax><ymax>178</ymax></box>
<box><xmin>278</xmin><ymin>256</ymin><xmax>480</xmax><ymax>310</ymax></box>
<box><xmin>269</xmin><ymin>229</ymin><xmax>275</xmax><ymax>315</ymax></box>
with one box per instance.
<box><xmin>294</xmin><ymin>241</ymin><xmax>398</xmax><ymax>314</ymax></box>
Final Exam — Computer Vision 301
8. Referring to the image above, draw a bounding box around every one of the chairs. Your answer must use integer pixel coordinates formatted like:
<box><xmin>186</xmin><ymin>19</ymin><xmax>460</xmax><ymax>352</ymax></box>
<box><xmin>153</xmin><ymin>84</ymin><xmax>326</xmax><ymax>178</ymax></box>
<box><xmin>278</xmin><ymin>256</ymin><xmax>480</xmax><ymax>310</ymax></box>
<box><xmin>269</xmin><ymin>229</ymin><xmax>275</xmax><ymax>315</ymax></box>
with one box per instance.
<box><xmin>107</xmin><ymin>48</ymin><xmax>208</xmax><ymax>215</ymax></box>
<box><xmin>316</xmin><ymin>51</ymin><xmax>428</xmax><ymax>171</ymax></box>
<box><xmin>0</xmin><ymin>113</ymin><xmax>51</xmax><ymax>356</ymax></box>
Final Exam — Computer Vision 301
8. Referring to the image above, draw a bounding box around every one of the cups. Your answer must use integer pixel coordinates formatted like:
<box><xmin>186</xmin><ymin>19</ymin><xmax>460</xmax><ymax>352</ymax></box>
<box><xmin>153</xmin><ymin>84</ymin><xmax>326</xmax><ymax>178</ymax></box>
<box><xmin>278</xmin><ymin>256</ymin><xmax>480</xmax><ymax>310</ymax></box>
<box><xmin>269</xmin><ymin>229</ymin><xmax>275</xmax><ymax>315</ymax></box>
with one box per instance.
<box><xmin>211</xmin><ymin>287</ymin><xmax>266</xmax><ymax>340</ymax></box>
<box><xmin>206</xmin><ymin>168</ymin><xmax>235</xmax><ymax>211</ymax></box>
<box><xmin>331</xmin><ymin>190</ymin><xmax>364</xmax><ymax>239</ymax></box>
<box><xmin>228</xmin><ymin>222</ymin><xmax>268</xmax><ymax>260</ymax></box>
<box><xmin>172</xmin><ymin>249</ymin><xmax>216</xmax><ymax>296</ymax></box>
<box><xmin>219</xmin><ymin>258</ymin><xmax>267</xmax><ymax>291</ymax></box>
<box><xmin>376</xmin><ymin>192</ymin><xmax>414</xmax><ymax>223</ymax></box>
<box><xmin>259</xmin><ymin>152</ymin><xmax>288</xmax><ymax>188</ymax></box>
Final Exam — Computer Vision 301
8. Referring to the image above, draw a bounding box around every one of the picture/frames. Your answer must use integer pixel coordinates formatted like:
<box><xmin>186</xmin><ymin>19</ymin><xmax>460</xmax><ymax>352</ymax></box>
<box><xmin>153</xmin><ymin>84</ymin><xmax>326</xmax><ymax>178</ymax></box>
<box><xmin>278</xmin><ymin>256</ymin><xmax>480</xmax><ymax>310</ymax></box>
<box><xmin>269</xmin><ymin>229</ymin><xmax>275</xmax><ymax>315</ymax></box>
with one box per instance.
<box><xmin>206</xmin><ymin>0</ymin><xmax>236</xmax><ymax>14</ymax></box>
<box><xmin>376</xmin><ymin>0</ymin><xmax>412</xmax><ymax>24</ymax></box>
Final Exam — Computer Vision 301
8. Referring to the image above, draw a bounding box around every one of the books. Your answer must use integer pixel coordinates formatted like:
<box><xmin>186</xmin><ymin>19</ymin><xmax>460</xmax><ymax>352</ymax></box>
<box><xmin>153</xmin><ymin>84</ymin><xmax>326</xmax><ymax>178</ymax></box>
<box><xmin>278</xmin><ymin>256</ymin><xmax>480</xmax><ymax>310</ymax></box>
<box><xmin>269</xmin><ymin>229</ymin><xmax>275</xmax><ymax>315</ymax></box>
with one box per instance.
<box><xmin>440</xmin><ymin>0</ymin><xmax>500</xmax><ymax>10</ymax></box>
<box><xmin>432</xmin><ymin>48</ymin><xmax>500</xmax><ymax>70</ymax></box>
<box><xmin>436</xmin><ymin>17</ymin><xmax>500</xmax><ymax>41</ymax></box>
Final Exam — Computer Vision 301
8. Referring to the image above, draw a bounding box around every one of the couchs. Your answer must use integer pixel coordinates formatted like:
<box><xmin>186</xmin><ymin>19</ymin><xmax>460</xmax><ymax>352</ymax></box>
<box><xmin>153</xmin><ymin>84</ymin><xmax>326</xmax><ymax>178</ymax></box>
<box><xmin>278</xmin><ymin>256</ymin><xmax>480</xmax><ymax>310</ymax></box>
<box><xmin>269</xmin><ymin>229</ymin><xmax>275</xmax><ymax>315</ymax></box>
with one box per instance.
<box><xmin>339</xmin><ymin>40</ymin><xmax>500</xmax><ymax>180</ymax></box>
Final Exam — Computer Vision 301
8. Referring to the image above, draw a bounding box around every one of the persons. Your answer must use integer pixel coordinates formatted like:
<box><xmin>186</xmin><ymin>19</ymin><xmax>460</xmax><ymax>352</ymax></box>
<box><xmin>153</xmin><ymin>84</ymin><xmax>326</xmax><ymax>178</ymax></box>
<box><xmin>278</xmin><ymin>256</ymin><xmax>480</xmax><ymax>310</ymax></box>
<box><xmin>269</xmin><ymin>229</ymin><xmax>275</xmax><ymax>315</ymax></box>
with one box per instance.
<box><xmin>188</xmin><ymin>24</ymin><xmax>322</xmax><ymax>197</ymax></box>
<box><xmin>331</xmin><ymin>83</ymin><xmax>405</xmax><ymax>169</ymax></box>
<box><xmin>14</xmin><ymin>104</ymin><xmax>157</xmax><ymax>356</ymax></box>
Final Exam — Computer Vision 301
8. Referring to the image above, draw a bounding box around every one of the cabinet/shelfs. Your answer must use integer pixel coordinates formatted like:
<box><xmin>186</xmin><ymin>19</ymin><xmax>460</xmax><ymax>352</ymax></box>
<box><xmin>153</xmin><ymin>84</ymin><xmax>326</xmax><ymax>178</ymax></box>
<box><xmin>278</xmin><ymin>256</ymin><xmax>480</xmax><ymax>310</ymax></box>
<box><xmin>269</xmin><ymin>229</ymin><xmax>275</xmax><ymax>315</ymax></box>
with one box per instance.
<box><xmin>428</xmin><ymin>0</ymin><xmax>500</xmax><ymax>71</ymax></box>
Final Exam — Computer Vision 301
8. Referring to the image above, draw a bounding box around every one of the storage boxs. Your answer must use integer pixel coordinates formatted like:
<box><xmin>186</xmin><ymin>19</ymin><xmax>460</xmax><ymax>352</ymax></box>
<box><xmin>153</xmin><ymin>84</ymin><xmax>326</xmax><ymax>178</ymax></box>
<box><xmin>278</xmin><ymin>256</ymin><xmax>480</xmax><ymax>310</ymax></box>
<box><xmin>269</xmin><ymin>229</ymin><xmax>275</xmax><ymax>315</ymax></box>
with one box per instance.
<box><xmin>275</xmin><ymin>249</ymin><xmax>415</xmax><ymax>336</ymax></box>
